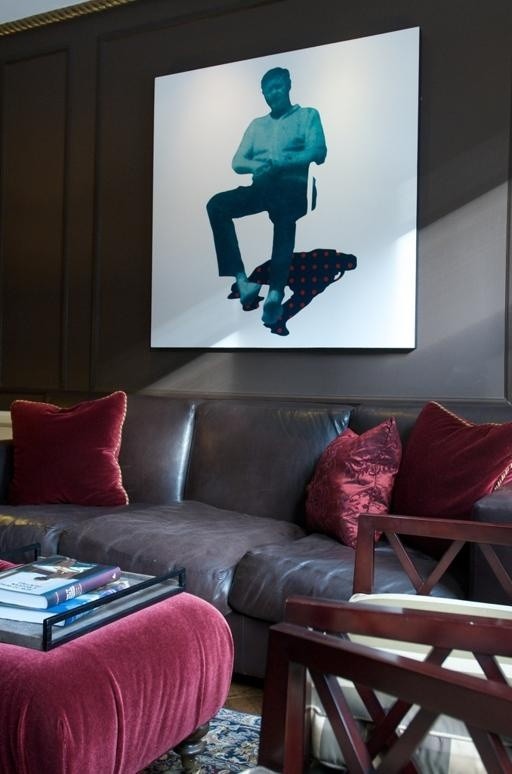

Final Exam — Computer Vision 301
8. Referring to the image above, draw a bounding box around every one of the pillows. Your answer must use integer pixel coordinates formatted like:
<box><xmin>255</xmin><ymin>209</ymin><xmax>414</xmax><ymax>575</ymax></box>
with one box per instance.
<box><xmin>395</xmin><ymin>399</ymin><xmax>511</xmax><ymax>521</ymax></box>
<box><xmin>299</xmin><ymin>415</ymin><xmax>405</xmax><ymax>547</ymax></box>
<box><xmin>9</xmin><ymin>389</ymin><xmax>129</xmax><ymax>510</ymax></box>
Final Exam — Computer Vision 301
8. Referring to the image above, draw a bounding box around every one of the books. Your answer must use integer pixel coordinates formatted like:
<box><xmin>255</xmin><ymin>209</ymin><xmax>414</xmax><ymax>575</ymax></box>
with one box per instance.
<box><xmin>1</xmin><ymin>553</ymin><xmax>186</xmax><ymax>652</ymax></box>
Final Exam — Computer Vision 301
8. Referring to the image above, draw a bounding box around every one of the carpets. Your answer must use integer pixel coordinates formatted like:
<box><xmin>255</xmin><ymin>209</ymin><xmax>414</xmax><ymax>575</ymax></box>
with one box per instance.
<box><xmin>133</xmin><ymin>705</ymin><xmax>260</xmax><ymax>774</ymax></box>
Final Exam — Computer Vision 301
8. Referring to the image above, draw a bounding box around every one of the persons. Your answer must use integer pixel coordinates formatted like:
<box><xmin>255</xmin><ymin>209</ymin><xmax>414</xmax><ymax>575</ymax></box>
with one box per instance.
<box><xmin>206</xmin><ymin>65</ymin><xmax>328</xmax><ymax>326</ymax></box>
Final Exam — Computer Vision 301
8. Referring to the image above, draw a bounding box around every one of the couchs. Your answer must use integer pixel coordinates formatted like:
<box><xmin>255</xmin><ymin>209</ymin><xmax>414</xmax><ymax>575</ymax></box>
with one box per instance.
<box><xmin>0</xmin><ymin>390</ymin><xmax>512</xmax><ymax>682</ymax></box>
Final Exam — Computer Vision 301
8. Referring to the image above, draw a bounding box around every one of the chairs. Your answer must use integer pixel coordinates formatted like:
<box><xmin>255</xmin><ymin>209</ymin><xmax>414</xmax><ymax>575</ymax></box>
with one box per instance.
<box><xmin>258</xmin><ymin>512</ymin><xmax>512</xmax><ymax>774</ymax></box>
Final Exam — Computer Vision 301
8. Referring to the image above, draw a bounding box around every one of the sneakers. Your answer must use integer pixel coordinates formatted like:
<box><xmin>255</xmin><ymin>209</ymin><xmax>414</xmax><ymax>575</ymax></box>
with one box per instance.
<box><xmin>260</xmin><ymin>289</ymin><xmax>284</xmax><ymax>326</ymax></box>
<box><xmin>237</xmin><ymin>281</ymin><xmax>261</xmax><ymax>307</ymax></box>
